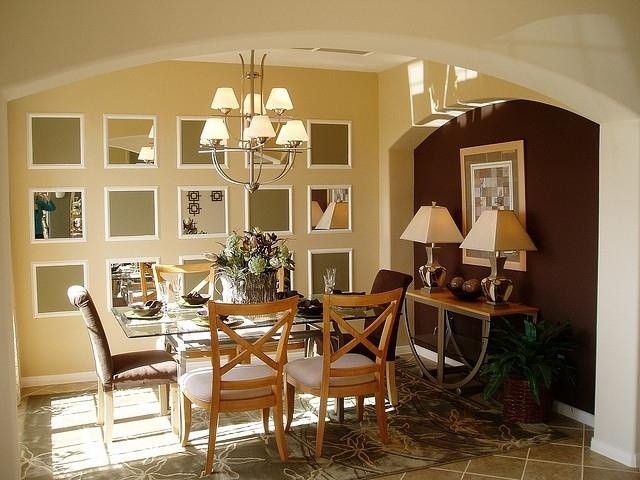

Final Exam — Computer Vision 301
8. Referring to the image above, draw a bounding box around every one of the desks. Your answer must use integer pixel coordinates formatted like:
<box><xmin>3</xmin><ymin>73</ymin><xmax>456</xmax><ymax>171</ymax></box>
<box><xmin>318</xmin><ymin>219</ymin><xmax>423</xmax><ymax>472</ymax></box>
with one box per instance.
<box><xmin>403</xmin><ymin>285</ymin><xmax>540</xmax><ymax>409</ymax></box>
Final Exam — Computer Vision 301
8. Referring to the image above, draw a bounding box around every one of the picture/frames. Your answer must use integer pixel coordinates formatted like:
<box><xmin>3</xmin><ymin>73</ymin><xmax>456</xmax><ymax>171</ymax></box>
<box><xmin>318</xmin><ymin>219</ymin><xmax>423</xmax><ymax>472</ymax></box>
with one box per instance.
<box><xmin>28</xmin><ymin>114</ymin><xmax>86</xmax><ymax>170</ymax></box>
<box><xmin>458</xmin><ymin>140</ymin><xmax>528</xmax><ymax>272</ymax></box>
<box><xmin>244</xmin><ymin>184</ymin><xmax>295</xmax><ymax>236</ymax></box>
<box><xmin>105</xmin><ymin>256</ymin><xmax>161</xmax><ymax>318</ymax></box>
<box><xmin>103</xmin><ymin>185</ymin><xmax>160</xmax><ymax>242</ymax></box>
<box><xmin>178</xmin><ymin>254</ymin><xmax>224</xmax><ymax>317</ymax></box>
<box><xmin>31</xmin><ymin>259</ymin><xmax>90</xmax><ymax>318</ymax></box>
<box><xmin>175</xmin><ymin>116</ymin><xmax>230</xmax><ymax>170</ymax></box>
<box><xmin>307</xmin><ymin>247</ymin><xmax>354</xmax><ymax>301</ymax></box>
<box><xmin>176</xmin><ymin>185</ymin><xmax>229</xmax><ymax>239</ymax></box>
<box><xmin>273</xmin><ymin>250</ymin><xmax>294</xmax><ymax>294</ymax></box>
<box><xmin>243</xmin><ymin>116</ymin><xmax>295</xmax><ymax>170</ymax></box>
<box><xmin>306</xmin><ymin>120</ymin><xmax>353</xmax><ymax>170</ymax></box>
<box><xmin>306</xmin><ymin>184</ymin><xmax>353</xmax><ymax>235</ymax></box>
<box><xmin>103</xmin><ymin>116</ymin><xmax>160</xmax><ymax>170</ymax></box>
<box><xmin>31</xmin><ymin>186</ymin><xmax>88</xmax><ymax>244</ymax></box>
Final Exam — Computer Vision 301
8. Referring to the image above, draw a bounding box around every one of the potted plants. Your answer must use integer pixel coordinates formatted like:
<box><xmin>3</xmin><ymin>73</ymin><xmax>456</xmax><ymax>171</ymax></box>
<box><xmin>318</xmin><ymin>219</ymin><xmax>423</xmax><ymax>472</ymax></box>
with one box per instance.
<box><xmin>476</xmin><ymin>314</ymin><xmax>584</xmax><ymax>423</ymax></box>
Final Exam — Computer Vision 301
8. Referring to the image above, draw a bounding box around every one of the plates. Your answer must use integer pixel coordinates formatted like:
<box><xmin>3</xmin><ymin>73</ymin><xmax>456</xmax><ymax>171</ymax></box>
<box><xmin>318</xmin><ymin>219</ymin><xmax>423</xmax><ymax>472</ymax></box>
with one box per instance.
<box><xmin>178</xmin><ymin>301</ymin><xmax>204</xmax><ymax>308</ymax></box>
<box><xmin>192</xmin><ymin>318</ymin><xmax>243</xmax><ymax>329</ymax></box>
<box><xmin>124</xmin><ymin>311</ymin><xmax>163</xmax><ymax>319</ymax></box>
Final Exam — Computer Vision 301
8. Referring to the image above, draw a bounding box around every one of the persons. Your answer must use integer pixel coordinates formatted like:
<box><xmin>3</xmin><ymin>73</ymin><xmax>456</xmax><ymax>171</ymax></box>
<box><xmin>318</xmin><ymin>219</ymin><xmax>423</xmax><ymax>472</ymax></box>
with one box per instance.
<box><xmin>34</xmin><ymin>192</ymin><xmax>56</xmax><ymax>239</ymax></box>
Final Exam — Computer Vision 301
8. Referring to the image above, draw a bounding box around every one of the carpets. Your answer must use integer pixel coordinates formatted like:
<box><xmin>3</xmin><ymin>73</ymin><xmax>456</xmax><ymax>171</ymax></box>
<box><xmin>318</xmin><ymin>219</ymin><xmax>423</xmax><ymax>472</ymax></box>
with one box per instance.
<box><xmin>22</xmin><ymin>353</ymin><xmax>572</xmax><ymax>480</ymax></box>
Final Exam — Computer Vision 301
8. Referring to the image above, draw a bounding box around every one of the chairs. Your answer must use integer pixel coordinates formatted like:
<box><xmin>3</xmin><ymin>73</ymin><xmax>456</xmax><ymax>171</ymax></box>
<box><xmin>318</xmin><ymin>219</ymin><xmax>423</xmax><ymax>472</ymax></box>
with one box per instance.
<box><xmin>284</xmin><ymin>287</ymin><xmax>404</xmax><ymax>460</ymax></box>
<box><xmin>316</xmin><ymin>269</ymin><xmax>413</xmax><ymax>423</ymax></box>
<box><xmin>149</xmin><ymin>263</ymin><xmax>249</xmax><ymax>414</ymax></box>
<box><xmin>67</xmin><ymin>285</ymin><xmax>180</xmax><ymax>442</ymax></box>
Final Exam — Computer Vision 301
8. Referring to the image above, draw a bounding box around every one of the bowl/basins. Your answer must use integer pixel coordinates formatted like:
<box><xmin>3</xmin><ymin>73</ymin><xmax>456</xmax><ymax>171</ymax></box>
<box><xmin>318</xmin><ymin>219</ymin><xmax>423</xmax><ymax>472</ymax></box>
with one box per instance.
<box><xmin>133</xmin><ymin>309</ymin><xmax>162</xmax><ymax>316</ymax></box>
<box><xmin>277</xmin><ymin>292</ymin><xmax>304</xmax><ymax>300</ymax></box>
<box><xmin>446</xmin><ymin>281</ymin><xmax>482</xmax><ymax>301</ymax></box>
<box><xmin>298</xmin><ymin>303</ymin><xmax>323</xmax><ymax>315</ymax></box>
<box><xmin>181</xmin><ymin>295</ymin><xmax>211</xmax><ymax>305</ymax></box>
<box><xmin>196</xmin><ymin>311</ymin><xmax>229</xmax><ymax>324</ymax></box>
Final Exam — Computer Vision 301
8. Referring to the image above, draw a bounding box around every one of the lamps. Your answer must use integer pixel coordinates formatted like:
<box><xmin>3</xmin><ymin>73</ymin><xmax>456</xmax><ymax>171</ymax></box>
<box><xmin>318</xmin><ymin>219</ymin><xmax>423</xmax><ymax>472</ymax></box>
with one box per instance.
<box><xmin>198</xmin><ymin>48</ymin><xmax>311</xmax><ymax>195</ymax></box>
<box><xmin>459</xmin><ymin>206</ymin><xmax>539</xmax><ymax>309</ymax></box>
<box><xmin>399</xmin><ymin>202</ymin><xmax>465</xmax><ymax>294</ymax></box>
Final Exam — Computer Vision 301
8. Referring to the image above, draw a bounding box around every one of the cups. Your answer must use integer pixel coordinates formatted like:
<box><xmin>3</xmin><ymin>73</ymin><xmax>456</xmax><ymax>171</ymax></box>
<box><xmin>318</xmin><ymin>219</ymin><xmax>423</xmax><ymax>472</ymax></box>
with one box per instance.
<box><xmin>326</xmin><ymin>267</ymin><xmax>336</xmax><ymax>288</ymax></box>
<box><xmin>323</xmin><ymin>275</ymin><xmax>334</xmax><ymax>294</ymax></box>
